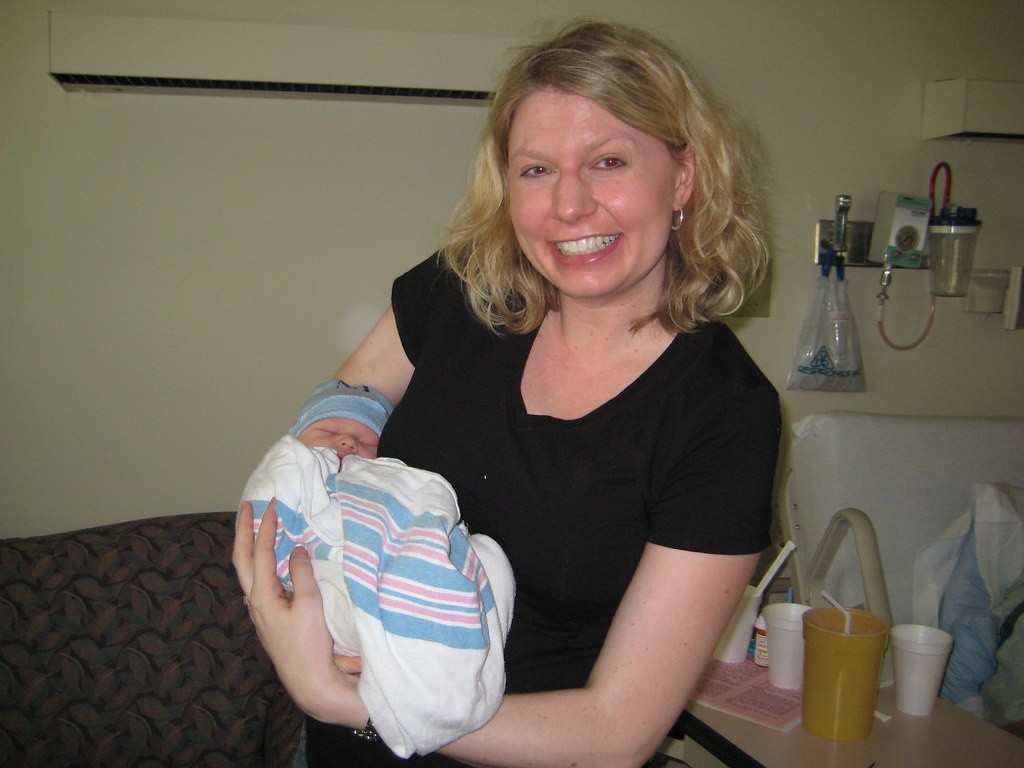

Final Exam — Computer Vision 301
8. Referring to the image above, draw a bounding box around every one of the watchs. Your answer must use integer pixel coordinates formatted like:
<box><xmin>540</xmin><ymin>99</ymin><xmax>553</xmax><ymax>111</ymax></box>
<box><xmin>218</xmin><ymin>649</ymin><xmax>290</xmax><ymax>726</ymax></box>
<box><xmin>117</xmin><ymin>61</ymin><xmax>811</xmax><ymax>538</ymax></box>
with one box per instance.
<box><xmin>351</xmin><ymin>718</ymin><xmax>381</xmax><ymax>743</ymax></box>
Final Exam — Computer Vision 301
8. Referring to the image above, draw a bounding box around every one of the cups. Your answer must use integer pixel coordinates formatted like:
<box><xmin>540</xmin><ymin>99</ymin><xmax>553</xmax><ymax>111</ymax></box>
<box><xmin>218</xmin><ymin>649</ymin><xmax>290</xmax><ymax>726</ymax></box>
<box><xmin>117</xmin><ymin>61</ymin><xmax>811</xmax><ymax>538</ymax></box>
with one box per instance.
<box><xmin>927</xmin><ymin>217</ymin><xmax>984</xmax><ymax>297</ymax></box>
<box><xmin>889</xmin><ymin>624</ymin><xmax>954</xmax><ymax>717</ymax></box>
<box><xmin>714</xmin><ymin>584</ymin><xmax>763</xmax><ymax>664</ymax></box>
<box><xmin>800</xmin><ymin>607</ymin><xmax>890</xmax><ymax>742</ymax></box>
<box><xmin>763</xmin><ymin>602</ymin><xmax>815</xmax><ymax>691</ymax></box>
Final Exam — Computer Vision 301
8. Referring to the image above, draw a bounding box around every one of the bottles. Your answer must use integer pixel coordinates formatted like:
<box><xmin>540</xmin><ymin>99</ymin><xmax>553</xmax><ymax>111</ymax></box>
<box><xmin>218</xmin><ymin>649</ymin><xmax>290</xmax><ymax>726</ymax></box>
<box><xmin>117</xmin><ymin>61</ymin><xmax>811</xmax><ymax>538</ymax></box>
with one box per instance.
<box><xmin>754</xmin><ymin>613</ymin><xmax>769</xmax><ymax>668</ymax></box>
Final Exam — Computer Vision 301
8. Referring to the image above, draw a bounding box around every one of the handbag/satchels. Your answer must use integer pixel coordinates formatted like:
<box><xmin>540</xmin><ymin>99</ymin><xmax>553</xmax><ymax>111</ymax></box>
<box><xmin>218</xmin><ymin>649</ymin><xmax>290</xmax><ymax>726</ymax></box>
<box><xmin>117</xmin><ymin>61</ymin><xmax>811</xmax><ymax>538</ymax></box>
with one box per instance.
<box><xmin>785</xmin><ymin>252</ymin><xmax>868</xmax><ymax>396</ymax></box>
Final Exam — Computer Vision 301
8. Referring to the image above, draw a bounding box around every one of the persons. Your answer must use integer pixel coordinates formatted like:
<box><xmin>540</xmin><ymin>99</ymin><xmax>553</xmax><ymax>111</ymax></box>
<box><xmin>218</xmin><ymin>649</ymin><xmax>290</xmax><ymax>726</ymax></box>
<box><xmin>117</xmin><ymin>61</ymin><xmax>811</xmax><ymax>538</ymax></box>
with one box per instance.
<box><xmin>231</xmin><ymin>15</ymin><xmax>779</xmax><ymax>768</ymax></box>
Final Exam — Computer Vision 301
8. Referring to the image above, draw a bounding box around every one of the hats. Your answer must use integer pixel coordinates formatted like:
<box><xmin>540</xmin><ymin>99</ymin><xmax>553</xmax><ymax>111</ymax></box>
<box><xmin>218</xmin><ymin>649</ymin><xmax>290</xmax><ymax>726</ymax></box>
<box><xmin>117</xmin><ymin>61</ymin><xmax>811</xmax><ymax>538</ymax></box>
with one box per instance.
<box><xmin>284</xmin><ymin>379</ymin><xmax>392</xmax><ymax>439</ymax></box>
